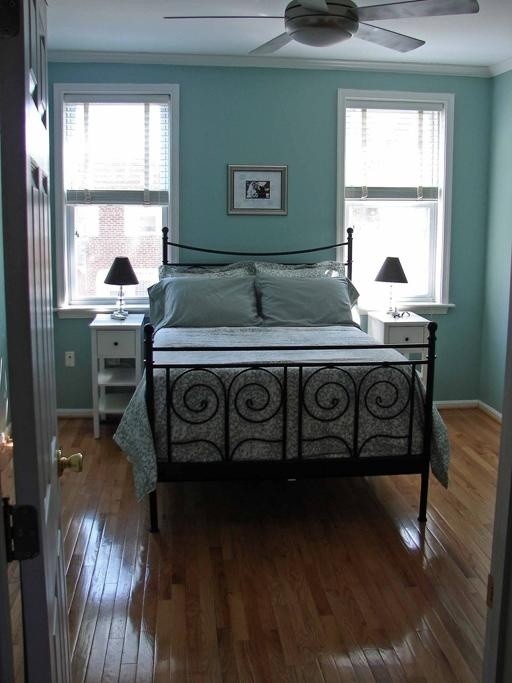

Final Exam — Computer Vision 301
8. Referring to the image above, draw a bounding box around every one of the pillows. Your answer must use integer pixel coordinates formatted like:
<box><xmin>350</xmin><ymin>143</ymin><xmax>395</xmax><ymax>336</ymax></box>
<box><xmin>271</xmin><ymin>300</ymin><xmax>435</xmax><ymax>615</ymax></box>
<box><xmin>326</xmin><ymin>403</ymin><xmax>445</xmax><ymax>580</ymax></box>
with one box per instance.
<box><xmin>159</xmin><ymin>276</ymin><xmax>257</xmax><ymax>324</ymax></box>
<box><xmin>254</xmin><ymin>261</ymin><xmax>345</xmax><ymax>278</ymax></box>
<box><xmin>157</xmin><ymin>261</ymin><xmax>254</xmax><ymax>280</ymax></box>
<box><xmin>256</xmin><ymin>273</ymin><xmax>351</xmax><ymax>326</ymax></box>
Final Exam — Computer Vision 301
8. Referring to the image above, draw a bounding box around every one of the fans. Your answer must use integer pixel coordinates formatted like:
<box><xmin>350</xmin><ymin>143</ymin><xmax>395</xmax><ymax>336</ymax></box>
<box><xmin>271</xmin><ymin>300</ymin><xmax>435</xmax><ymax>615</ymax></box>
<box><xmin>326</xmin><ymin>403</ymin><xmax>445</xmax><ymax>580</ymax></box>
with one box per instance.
<box><xmin>163</xmin><ymin>0</ymin><xmax>480</xmax><ymax>55</ymax></box>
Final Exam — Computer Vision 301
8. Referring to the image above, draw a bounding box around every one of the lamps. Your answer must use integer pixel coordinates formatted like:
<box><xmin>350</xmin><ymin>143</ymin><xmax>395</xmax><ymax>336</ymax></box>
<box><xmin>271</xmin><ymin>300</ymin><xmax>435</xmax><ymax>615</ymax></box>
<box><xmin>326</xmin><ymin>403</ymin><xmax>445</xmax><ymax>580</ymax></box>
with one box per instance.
<box><xmin>103</xmin><ymin>256</ymin><xmax>139</xmax><ymax>320</ymax></box>
<box><xmin>290</xmin><ymin>29</ymin><xmax>351</xmax><ymax>48</ymax></box>
<box><xmin>373</xmin><ymin>255</ymin><xmax>409</xmax><ymax>313</ymax></box>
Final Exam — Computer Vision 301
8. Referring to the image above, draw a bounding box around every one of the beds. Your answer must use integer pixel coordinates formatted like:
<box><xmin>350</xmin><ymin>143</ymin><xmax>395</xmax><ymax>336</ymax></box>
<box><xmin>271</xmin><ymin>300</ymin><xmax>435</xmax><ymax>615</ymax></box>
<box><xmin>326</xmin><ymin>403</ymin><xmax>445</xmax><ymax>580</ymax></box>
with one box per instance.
<box><xmin>140</xmin><ymin>226</ymin><xmax>437</xmax><ymax>537</ymax></box>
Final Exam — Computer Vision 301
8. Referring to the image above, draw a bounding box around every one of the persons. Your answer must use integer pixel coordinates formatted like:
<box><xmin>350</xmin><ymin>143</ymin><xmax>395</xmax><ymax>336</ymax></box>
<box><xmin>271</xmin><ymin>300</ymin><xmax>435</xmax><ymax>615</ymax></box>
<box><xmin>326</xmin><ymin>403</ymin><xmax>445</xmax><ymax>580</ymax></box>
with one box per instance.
<box><xmin>252</xmin><ymin>183</ymin><xmax>266</xmax><ymax>198</ymax></box>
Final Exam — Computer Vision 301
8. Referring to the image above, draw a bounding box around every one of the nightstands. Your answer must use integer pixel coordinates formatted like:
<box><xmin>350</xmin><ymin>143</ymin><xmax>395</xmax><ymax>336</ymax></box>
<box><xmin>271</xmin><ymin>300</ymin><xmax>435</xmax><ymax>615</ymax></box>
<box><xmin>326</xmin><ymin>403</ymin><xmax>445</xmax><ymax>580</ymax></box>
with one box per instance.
<box><xmin>366</xmin><ymin>311</ymin><xmax>432</xmax><ymax>392</ymax></box>
<box><xmin>88</xmin><ymin>313</ymin><xmax>145</xmax><ymax>438</ymax></box>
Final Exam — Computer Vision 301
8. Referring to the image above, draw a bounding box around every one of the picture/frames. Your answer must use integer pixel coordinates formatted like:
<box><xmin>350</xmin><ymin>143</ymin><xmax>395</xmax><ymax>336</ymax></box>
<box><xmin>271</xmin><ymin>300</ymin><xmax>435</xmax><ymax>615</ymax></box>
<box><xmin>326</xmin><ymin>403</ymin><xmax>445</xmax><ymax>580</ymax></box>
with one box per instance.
<box><xmin>226</xmin><ymin>164</ymin><xmax>288</xmax><ymax>216</ymax></box>
